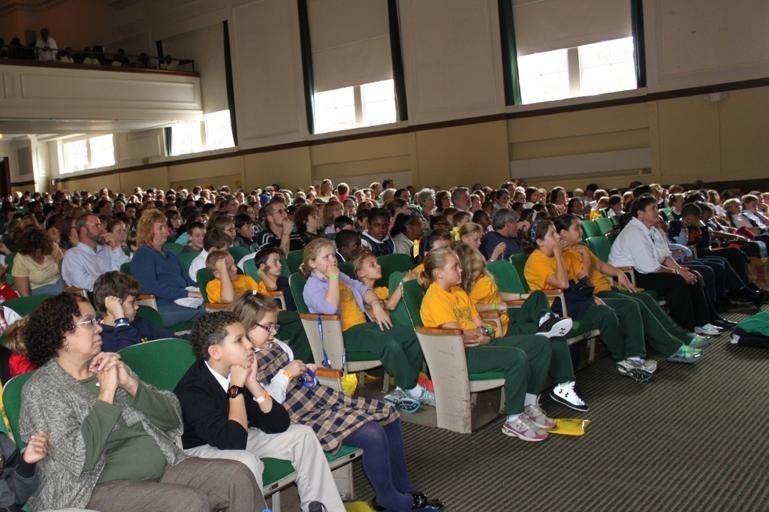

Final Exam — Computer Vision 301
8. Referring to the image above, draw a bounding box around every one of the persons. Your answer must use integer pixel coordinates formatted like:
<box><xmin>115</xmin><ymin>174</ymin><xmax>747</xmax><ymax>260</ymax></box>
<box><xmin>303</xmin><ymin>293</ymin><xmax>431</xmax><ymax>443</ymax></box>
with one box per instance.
<box><xmin>0</xmin><ymin>28</ymin><xmax>182</xmax><ymax>72</ymax></box>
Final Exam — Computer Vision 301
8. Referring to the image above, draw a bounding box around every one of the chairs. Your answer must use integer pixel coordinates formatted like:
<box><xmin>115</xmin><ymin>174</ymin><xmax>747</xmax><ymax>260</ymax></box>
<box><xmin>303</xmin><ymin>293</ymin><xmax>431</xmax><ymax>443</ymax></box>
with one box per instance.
<box><xmin>288</xmin><ymin>207</ymin><xmax>673</xmax><ymax>434</ymax></box>
<box><xmin>0</xmin><ymin>207</ymin><xmax>367</xmax><ymax>512</ymax></box>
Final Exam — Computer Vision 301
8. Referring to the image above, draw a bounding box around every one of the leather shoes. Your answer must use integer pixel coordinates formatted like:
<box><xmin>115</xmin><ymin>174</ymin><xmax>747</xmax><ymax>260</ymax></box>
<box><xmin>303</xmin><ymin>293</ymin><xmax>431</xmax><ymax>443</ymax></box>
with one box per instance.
<box><xmin>712</xmin><ymin>315</ymin><xmax>738</xmax><ymax>327</ymax></box>
<box><xmin>372</xmin><ymin>493</ymin><xmax>444</xmax><ymax>512</ymax></box>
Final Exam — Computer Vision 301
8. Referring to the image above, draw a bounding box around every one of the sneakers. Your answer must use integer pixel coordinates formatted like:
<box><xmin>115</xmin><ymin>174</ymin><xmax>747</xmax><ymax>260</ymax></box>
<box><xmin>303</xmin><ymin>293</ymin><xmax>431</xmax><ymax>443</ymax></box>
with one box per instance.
<box><xmin>641</xmin><ymin>359</ymin><xmax>658</xmax><ymax>373</ymax></box>
<box><xmin>550</xmin><ymin>381</ymin><xmax>590</xmax><ymax>413</ymax></box>
<box><xmin>416</xmin><ymin>390</ymin><xmax>438</xmax><ymax>409</ymax></box>
<box><xmin>501</xmin><ymin>414</ymin><xmax>549</xmax><ymax>443</ymax></box>
<box><xmin>616</xmin><ymin>358</ymin><xmax>653</xmax><ymax>382</ymax></box>
<box><xmin>666</xmin><ymin>344</ymin><xmax>704</xmax><ymax>364</ymax></box>
<box><xmin>729</xmin><ymin>332</ymin><xmax>740</xmax><ymax>344</ymax></box>
<box><xmin>384</xmin><ymin>390</ymin><xmax>420</xmax><ymax>414</ymax></box>
<box><xmin>687</xmin><ymin>335</ymin><xmax>710</xmax><ymax>349</ymax></box>
<box><xmin>536</xmin><ymin>313</ymin><xmax>573</xmax><ymax>339</ymax></box>
<box><xmin>729</xmin><ymin>283</ymin><xmax>769</xmax><ymax>305</ymax></box>
<box><xmin>523</xmin><ymin>403</ymin><xmax>557</xmax><ymax>431</ymax></box>
<box><xmin>693</xmin><ymin>323</ymin><xmax>723</xmax><ymax>336</ymax></box>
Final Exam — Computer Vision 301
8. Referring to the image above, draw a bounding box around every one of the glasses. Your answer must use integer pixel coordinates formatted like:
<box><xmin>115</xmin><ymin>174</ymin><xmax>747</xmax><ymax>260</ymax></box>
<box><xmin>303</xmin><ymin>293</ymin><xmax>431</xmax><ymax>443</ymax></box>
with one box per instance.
<box><xmin>73</xmin><ymin>319</ymin><xmax>103</xmax><ymax>328</ymax></box>
<box><xmin>253</xmin><ymin>322</ymin><xmax>281</xmax><ymax>331</ymax></box>
